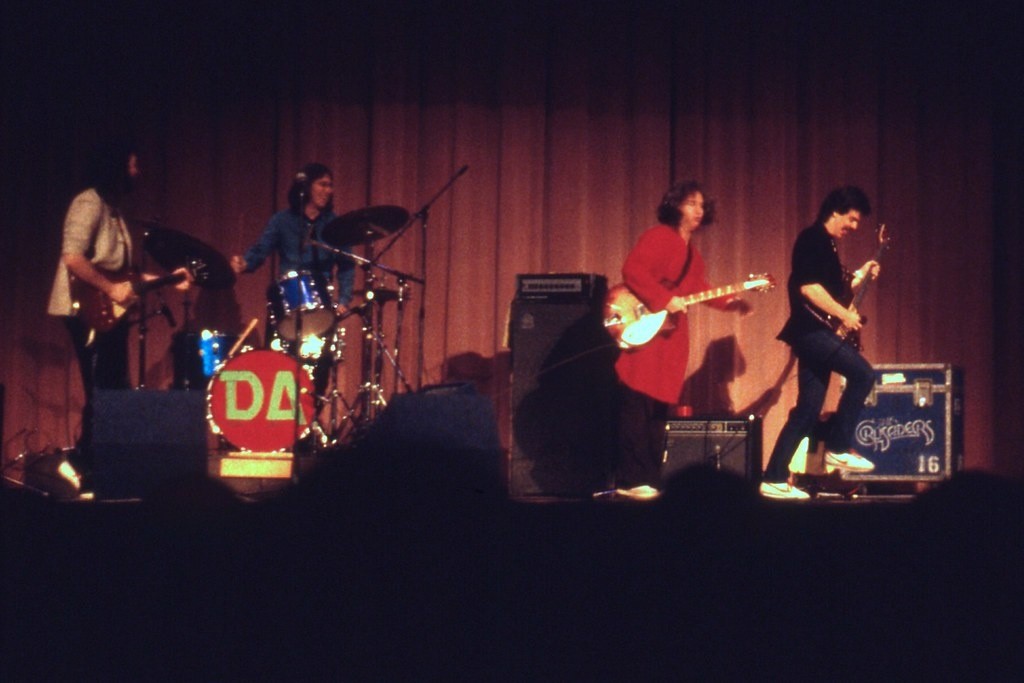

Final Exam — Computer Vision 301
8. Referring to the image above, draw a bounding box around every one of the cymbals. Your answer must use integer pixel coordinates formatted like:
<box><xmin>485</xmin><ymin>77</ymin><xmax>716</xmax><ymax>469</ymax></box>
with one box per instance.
<box><xmin>142</xmin><ymin>227</ymin><xmax>236</xmax><ymax>291</ymax></box>
<box><xmin>352</xmin><ymin>286</ymin><xmax>409</xmax><ymax>303</ymax></box>
<box><xmin>319</xmin><ymin>203</ymin><xmax>410</xmax><ymax>246</ymax></box>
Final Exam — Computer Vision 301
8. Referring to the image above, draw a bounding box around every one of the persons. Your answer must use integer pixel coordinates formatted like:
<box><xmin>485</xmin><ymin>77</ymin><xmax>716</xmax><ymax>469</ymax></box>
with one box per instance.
<box><xmin>47</xmin><ymin>140</ymin><xmax>195</xmax><ymax>500</ymax></box>
<box><xmin>758</xmin><ymin>186</ymin><xmax>881</xmax><ymax>502</ymax></box>
<box><xmin>229</xmin><ymin>160</ymin><xmax>358</xmax><ymax>399</ymax></box>
<box><xmin>603</xmin><ymin>179</ymin><xmax>743</xmax><ymax>501</ymax></box>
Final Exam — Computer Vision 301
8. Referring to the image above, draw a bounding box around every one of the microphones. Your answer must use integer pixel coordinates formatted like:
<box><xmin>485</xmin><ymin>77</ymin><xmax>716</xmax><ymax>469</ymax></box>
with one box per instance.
<box><xmin>302</xmin><ymin>223</ymin><xmax>314</xmax><ymax>245</ymax></box>
<box><xmin>296</xmin><ymin>172</ymin><xmax>306</xmax><ymax>195</ymax></box>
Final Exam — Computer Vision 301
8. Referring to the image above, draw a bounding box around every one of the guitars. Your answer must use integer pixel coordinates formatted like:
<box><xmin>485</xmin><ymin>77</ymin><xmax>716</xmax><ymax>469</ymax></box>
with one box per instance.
<box><xmin>69</xmin><ymin>260</ymin><xmax>207</xmax><ymax>333</ymax></box>
<box><xmin>599</xmin><ymin>272</ymin><xmax>778</xmax><ymax>352</ymax></box>
<box><xmin>828</xmin><ymin>223</ymin><xmax>888</xmax><ymax>351</ymax></box>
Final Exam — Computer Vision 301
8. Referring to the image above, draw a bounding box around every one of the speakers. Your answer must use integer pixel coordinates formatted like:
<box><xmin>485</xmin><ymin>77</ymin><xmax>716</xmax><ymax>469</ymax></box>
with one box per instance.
<box><xmin>506</xmin><ymin>300</ymin><xmax>620</xmax><ymax>500</ymax></box>
<box><xmin>661</xmin><ymin>415</ymin><xmax>753</xmax><ymax>496</ymax></box>
<box><xmin>87</xmin><ymin>388</ymin><xmax>207</xmax><ymax>501</ymax></box>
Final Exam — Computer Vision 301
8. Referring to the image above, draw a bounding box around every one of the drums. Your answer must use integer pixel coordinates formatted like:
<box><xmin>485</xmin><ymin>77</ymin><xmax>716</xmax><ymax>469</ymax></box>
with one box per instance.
<box><xmin>205</xmin><ymin>347</ymin><xmax>317</xmax><ymax>452</ymax></box>
<box><xmin>170</xmin><ymin>327</ymin><xmax>238</xmax><ymax>391</ymax></box>
<box><xmin>265</xmin><ymin>268</ymin><xmax>333</xmax><ymax>320</ymax></box>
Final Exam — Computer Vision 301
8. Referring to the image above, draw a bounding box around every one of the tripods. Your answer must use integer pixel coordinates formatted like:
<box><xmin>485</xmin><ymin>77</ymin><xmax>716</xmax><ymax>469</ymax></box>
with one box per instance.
<box><xmin>306</xmin><ymin>235</ymin><xmax>390</xmax><ymax>456</ymax></box>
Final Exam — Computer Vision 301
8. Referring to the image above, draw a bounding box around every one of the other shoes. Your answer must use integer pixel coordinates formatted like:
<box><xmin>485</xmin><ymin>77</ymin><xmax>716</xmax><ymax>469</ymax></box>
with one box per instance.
<box><xmin>760</xmin><ymin>482</ymin><xmax>810</xmax><ymax>500</ymax></box>
<box><xmin>616</xmin><ymin>484</ymin><xmax>659</xmax><ymax>499</ymax></box>
<box><xmin>57</xmin><ymin>461</ymin><xmax>95</xmax><ymax>501</ymax></box>
<box><xmin>824</xmin><ymin>448</ymin><xmax>875</xmax><ymax>473</ymax></box>
